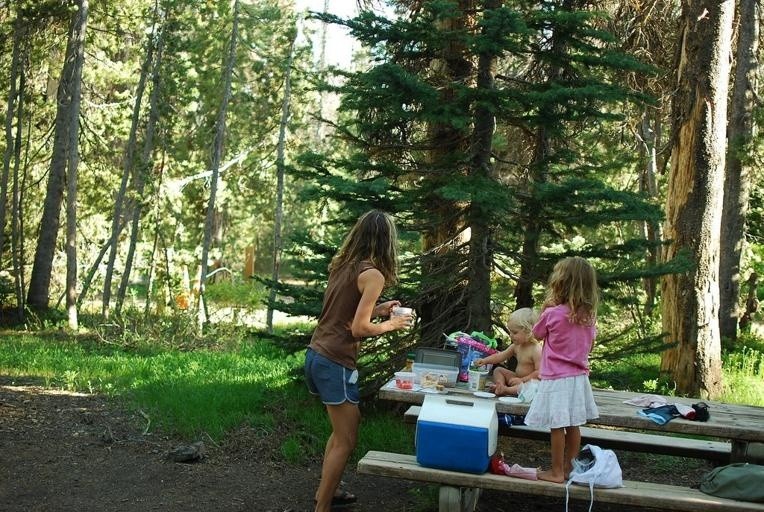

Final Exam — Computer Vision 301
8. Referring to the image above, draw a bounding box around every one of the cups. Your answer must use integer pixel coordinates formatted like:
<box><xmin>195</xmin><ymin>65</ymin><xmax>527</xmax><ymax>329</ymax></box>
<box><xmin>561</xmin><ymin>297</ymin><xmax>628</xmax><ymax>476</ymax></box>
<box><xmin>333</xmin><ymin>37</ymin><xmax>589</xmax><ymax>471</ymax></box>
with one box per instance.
<box><xmin>394</xmin><ymin>308</ymin><xmax>411</xmax><ymax>325</ymax></box>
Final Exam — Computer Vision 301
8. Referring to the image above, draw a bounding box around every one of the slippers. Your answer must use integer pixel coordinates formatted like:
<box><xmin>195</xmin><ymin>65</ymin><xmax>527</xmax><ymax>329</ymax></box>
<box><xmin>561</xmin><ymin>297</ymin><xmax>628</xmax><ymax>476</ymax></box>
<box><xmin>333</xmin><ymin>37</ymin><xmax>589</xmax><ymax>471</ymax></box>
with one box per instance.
<box><xmin>314</xmin><ymin>489</ymin><xmax>357</xmax><ymax>505</ymax></box>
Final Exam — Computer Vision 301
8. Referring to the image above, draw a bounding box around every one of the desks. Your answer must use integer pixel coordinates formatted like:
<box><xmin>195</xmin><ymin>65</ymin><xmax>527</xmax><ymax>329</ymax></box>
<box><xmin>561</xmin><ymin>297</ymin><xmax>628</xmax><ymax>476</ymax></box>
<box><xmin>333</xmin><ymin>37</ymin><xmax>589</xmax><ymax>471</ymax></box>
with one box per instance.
<box><xmin>379</xmin><ymin>365</ymin><xmax>764</xmax><ymax>512</ymax></box>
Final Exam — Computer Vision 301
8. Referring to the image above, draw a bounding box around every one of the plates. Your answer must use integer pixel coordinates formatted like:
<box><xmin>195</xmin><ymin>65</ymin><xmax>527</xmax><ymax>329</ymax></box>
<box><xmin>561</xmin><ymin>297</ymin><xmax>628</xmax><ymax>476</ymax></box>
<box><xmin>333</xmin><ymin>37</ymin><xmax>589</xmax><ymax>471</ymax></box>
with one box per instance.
<box><xmin>473</xmin><ymin>391</ymin><xmax>496</xmax><ymax>398</ymax></box>
<box><xmin>499</xmin><ymin>396</ymin><xmax>521</xmax><ymax>404</ymax></box>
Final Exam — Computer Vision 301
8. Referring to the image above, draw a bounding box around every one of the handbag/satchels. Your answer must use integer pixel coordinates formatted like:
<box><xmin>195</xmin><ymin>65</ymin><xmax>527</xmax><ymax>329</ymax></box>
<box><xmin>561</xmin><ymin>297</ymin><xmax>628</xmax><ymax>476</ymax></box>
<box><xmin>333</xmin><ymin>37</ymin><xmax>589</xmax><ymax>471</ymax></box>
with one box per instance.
<box><xmin>568</xmin><ymin>444</ymin><xmax>622</xmax><ymax>489</ymax></box>
<box><xmin>457</xmin><ymin>336</ymin><xmax>497</xmax><ymax>382</ymax></box>
<box><xmin>691</xmin><ymin>463</ymin><xmax>764</xmax><ymax>503</ymax></box>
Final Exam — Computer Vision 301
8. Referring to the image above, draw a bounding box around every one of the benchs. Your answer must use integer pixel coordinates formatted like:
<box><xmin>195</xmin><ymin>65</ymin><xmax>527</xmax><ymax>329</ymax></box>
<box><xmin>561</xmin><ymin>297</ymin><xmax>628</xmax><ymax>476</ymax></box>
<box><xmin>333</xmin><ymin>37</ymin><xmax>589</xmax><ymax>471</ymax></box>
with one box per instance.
<box><xmin>354</xmin><ymin>449</ymin><xmax>764</xmax><ymax>512</ymax></box>
<box><xmin>404</xmin><ymin>405</ymin><xmax>735</xmax><ymax>487</ymax></box>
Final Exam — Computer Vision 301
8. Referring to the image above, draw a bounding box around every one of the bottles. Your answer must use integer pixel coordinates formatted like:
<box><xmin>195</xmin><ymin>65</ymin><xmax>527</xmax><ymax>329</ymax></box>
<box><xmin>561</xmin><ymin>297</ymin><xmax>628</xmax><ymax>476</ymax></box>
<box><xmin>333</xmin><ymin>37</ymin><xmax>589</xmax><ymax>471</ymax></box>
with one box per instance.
<box><xmin>405</xmin><ymin>353</ymin><xmax>416</xmax><ymax>372</ymax></box>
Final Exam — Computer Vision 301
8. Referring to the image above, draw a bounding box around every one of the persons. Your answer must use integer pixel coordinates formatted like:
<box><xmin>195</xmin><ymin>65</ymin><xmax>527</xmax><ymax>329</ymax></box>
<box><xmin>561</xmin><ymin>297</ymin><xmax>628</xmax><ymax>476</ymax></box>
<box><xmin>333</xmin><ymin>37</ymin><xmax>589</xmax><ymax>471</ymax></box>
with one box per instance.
<box><xmin>303</xmin><ymin>210</ymin><xmax>412</xmax><ymax>510</ymax></box>
<box><xmin>522</xmin><ymin>256</ymin><xmax>600</xmax><ymax>482</ymax></box>
<box><xmin>475</xmin><ymin>308</ymin><xmax>541</xmax><ymax>395</ymax></box>
